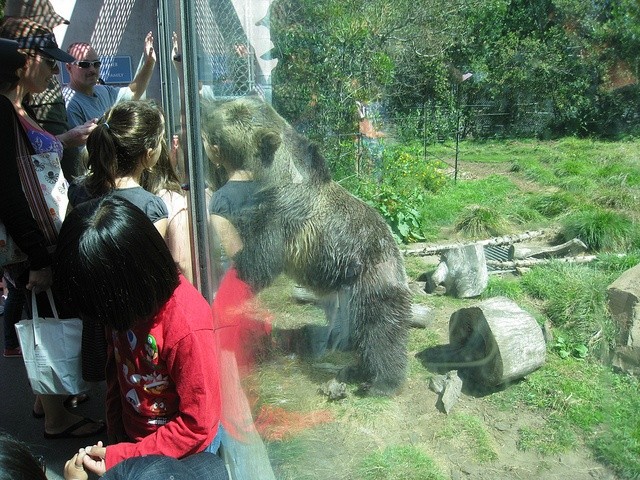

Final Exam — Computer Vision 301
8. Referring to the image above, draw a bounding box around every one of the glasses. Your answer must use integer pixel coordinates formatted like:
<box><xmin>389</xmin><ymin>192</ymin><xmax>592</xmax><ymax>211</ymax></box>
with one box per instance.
<box><xmin>71</xmin><ymin>60</ymin><xmax>101</xmax><ymax>69</ymax></box>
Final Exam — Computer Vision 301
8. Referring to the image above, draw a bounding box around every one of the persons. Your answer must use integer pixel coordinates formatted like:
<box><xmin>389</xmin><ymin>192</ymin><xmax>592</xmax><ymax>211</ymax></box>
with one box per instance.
<box><xmin>51</xmin><ymin>194</ymin><xmax>221</xmax><ymax>477</ymax></box>
<box><xmin>4</xmin><ymin>1</ymin><xmax>99</xmax><ymax>181</ymax></box>
<box><xmin>0</xmin><ymin>433</ymin><xmax>229</xmax><ymax>480</ymax></box>
<box><xmin>203</xmin><ymin>99</ymin><xmax>258</xmax><ymax>257</ymax></box>
<box><xmin>60</xmin><ymin>31</ymin><xmax>157</xmax><ymax>176</ymax></box>
<box><xmin>139</xmin><ymin>136</ymin><xmax>193</xmax><ymax>288</ymax></box>
<box><xmin>0</xmin><ymin>16</ymin><xmax>107</xmax><ymax>439</ymax></box>
<box><xmin>65</xmin><ymin>100</ymin><xmax>169</xmax><ymax>241</ymax></box>
<box><xmin>212</xmin><ymin>242</ymin><xmax>290</xmax><ymax>480</ymax></box>
<box><xmin>173</xmin><ymin>131</ymin><xmax>190</xmax><ymax>188</ymax></box>
<box><xmin>171</xmin><ymin>33</ymin><xmax>213</xmax><ymax>130</ymax></box>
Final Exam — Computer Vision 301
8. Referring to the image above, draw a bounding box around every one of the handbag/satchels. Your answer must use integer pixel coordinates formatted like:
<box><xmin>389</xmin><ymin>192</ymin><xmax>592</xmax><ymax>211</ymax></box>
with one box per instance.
<box><xmin>11</xmin><ymin>117</ymin><xmax>70</xmax><ymax>238</ymax></box>
<box><xmin>14</xmin><ymin>282</ymin><xmax>83</xmax><ymax>396</ymax></box>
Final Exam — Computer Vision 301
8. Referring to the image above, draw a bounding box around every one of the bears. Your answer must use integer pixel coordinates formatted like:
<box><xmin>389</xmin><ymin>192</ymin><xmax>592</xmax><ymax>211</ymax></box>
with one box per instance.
<box><xmin>199</xmin><ymin>89</ymin><xmax>414</xmax><ymax>400</ymax></box>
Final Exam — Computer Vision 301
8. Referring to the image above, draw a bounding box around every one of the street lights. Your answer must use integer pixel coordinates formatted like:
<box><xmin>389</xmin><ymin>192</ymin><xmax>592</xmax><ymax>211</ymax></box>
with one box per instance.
<box><xmin>455</xmin><ymin>73</ymin><xmax>473</xmax><ymax>184</ymax></box>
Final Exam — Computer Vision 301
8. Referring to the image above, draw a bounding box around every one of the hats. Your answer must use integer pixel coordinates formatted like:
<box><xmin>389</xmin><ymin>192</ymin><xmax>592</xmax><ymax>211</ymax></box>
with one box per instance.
<box><xmin>1</xmin><ymin>33</ymin><xmax>76</xmax><ymax>62</ymax></box>
